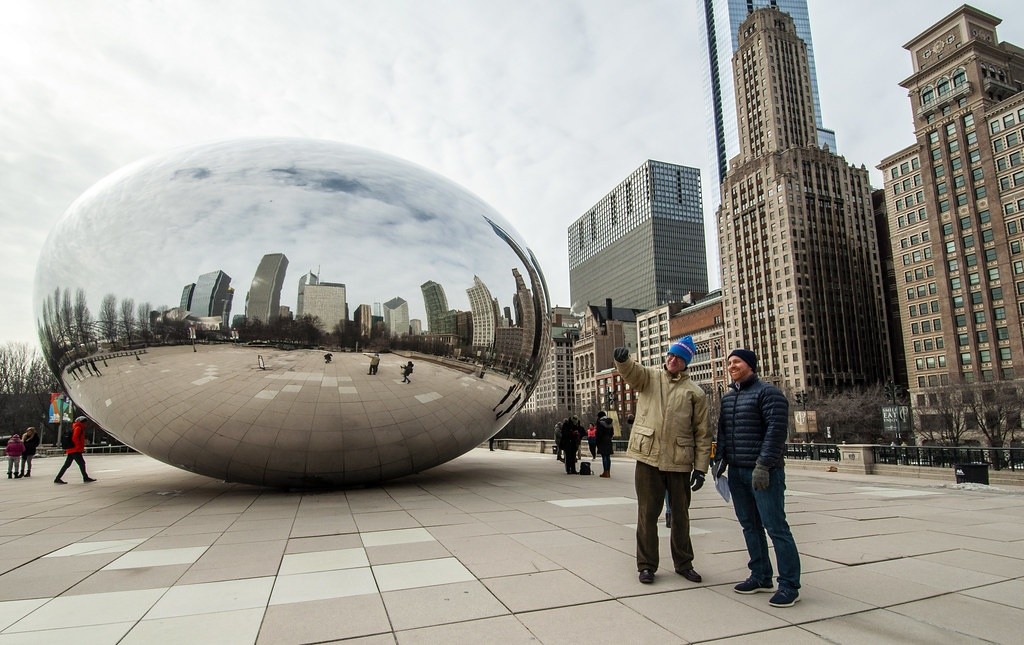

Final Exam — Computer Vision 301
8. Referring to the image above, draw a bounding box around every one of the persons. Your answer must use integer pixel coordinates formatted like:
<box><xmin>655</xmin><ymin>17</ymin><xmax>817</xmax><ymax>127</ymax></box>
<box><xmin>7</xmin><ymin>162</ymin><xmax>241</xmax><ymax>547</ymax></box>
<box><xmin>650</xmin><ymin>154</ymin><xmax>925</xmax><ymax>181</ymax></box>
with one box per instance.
<box><xmin>364</xmin><ymin>353</ymin><xmax>380</xmax><ymax>375</ymax></box>
<box><xmin>716</xmin><ymin>349</ymin><xmax>802</xmax><ymax>606</ymax></box>
<box><xmin>54</xmin><ymin>416</ymin><xmax>97</xmax><ymax>483</ymax></box>
<box><xmin>612</xmin><ymin>336</ymin><xmax>713</xmax><ymax>583</ymax></box>
<box><xmin>72</xmin><ymin>360</ymin><xmax>102</xmax><ymax>376</ymax></box>
<box><xmin>586</xmin><ymin>423</ymin><xmax>597</xmax><ymax>459</ymax></box>
<box><xmin>324</xmin><ymin>353</ymin><xmax>332</xmax><ymax>363</ymax></box>
<box><xmin>493</xmin><ymin>385</ymin><xmax>521</xmax><ymax>420</ymax></box>
<box><xmin>5</xmin><ymin>434</ymin><xmax>25</xmax><ymax>479</ymax></box>
<box><xmin>489</xmin><ymin>437</ymin><xmax>494</xmax><ymax>451</ymax></box>
<box><xmin>596</xmin><ymin>412</ymin><xmax>614</xmax><ymax>478</ymax></box>
<box><xmin>19</xmin><ymin>427</ymin><xmax>40</xmax><ymax>477</ymax></box>
<box><xmin>664</xmin><ymin>490</ymin><xmax>672</xmax><ymax>528</ymax></box>
<box><xmin>401</xmin><ymin>361</ymin><xmax>413</xmax><ymax>383</ymax></box>
<box><xmin>553</xmin><ymin>414</ymin><xmax>586</xmax><ymax>474</ymax></box>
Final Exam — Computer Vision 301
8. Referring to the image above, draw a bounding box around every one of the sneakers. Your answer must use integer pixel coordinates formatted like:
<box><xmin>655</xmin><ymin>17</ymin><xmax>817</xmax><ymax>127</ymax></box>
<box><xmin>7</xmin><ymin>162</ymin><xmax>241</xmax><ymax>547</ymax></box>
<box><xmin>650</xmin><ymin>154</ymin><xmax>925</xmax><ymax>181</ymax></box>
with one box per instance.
<box><xmin>768</xmin><ymin>584</ymin><xmax>801</xmax><ymax>608</ymax></box>
<box><xmin>733</xmin><ymin>575</ymin><xmax>775</xmax><ymax>595</ymax></box>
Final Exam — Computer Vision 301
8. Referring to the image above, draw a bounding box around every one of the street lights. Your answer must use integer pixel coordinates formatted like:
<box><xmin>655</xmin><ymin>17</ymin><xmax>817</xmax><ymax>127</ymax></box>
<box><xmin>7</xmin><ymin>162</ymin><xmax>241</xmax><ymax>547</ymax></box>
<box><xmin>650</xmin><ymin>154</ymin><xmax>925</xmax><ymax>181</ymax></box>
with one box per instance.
<box><xmin>795</xmin><ymin>390</ymin><xmax>814</xmax><ymax>459</ymax></box>
<box><xmin>885</xmin><ymin>379</ymin><xmax>909</xmax><ymax>465</ymax></box>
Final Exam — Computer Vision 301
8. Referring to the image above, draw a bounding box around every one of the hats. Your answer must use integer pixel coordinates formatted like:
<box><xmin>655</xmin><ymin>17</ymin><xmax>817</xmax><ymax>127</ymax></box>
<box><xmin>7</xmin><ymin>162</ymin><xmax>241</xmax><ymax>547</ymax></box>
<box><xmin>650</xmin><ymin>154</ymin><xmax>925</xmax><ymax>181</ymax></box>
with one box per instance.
<box><xmin>597</xmin><ymin>411</ymin><xmax>606</xmax><ymax>418</ymax></box>
<box><xmin>76</xmin><ymin>416</ymin><xmax>88</xmax><ymax>423</ymax></box>
<box><xmin>667</xmin><ymin>335</ymin><xmax>697</xmax><ymax>367</ymax></box>
<box><xmin>12</xmin><ymin>434</ymin><xmax>19</xmax><ymax>438</ymax></box>
<box><xmin>408</xmin><ymin>361</ymin><xmax>412</xmax><ymax>363</ymax></box>
<box><xmin>728</xmin><ymin>348</ymin><xmax>757</xmax><ymax>373</ymax></box>
<box><xmin>375</xmin><ymin>353</ymin><xmax>379</xmax><ymax>357</ymax></box>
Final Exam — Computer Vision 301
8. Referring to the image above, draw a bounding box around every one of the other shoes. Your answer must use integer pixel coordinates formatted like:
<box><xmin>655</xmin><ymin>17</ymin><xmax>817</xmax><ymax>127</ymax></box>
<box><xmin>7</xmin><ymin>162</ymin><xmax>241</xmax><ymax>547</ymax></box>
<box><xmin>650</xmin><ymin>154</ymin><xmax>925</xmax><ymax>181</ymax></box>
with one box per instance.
<box><xmin>639</xmin><ymin>569</ymin><xmax>654</xmax><ymax>584</ymax></box>
<box><xmin>54</xmin><ymin>478</ymin><xmax>67</xmax><ymax>484</ymax></box>
<box><xmin>367</xmin><ymin>373</ymin><xmax>375</xmax><ymax>375</ymax></box>
<box><xmin>600</xmin><ymin>471</ymin><xmax>610</xmax><ymax>478</ymax></box>
<box><xmin>592</xmin><ymin>457</ymin><xmax>597</xmax><ymax>459</ymax></box>
<box><xmin>84</xmin><ymin>478</ymin><xmax>96</xmax><ymax>482</ymax></box>
<box><xmin>567</xmin><ymin>470</ymin><xmax>579</xmax><ymax>474</ymax></box>
<box><xmin>557</xmin><ymin>458</ymin><xmax>565</xmax><ymax>463</ymax></box>
<box><xmin>675</xmin><ymin>568</ymin><xmax>701</xmax><ymax>582</ymax></box>
<box><xmin>401</xmin><ymin>380</ymin><xmax>411</xmax><ymax>384</ymax></box>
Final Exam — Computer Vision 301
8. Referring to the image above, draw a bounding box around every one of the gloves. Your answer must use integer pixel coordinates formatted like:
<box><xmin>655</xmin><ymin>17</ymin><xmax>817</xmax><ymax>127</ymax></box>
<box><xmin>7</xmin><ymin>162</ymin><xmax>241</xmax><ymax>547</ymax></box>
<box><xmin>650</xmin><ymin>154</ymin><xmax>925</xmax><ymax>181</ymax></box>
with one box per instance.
<box><xmin>715</xmin><ymin>459</ymin><xmax>727</xmax><ymax>478</ymax></box>
<box><xmin>628</xmin><ymin>413</ymin><xmax>635</xmax><ymax>420</ymax></box>
<box><xmin>613</xmin><ymin>347</ymin><xmax>629</xmax><ymax>363</ymax></box>
<box><xmin>751</xmin><ymin>460</ymin><xmax>772</xmax><ymax>491</ymax></box>
<box><xmin>690</xmin><ymin>470</ymin><xmax>706</xmax><ymax>491</ymax></box>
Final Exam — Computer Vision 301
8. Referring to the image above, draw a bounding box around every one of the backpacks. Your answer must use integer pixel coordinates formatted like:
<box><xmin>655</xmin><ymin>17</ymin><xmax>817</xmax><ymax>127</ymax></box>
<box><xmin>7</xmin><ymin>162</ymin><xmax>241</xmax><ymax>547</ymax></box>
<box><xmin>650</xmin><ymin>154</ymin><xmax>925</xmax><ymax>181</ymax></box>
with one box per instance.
<box><xmin>61</xmin><ymin>426</ymin><xmax>74</xmax><ymax>449</ymax></box>
<box><xmin>580</xmin><ymin>462</ymin><xmax>591</xmax><ymax>475</ymax></box>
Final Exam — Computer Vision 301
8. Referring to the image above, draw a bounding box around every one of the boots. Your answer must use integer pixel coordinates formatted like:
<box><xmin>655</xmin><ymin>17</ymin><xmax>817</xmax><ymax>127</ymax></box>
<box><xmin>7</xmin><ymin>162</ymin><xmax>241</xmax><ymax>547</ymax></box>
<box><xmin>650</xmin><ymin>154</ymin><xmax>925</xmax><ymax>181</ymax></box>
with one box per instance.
<box><xmin>17</xmin><ymin>470</ymin><xmax>31</xmax><ymax>478</ymax></box>
<box><xmin>7</xmin><ymin>471</ymin><xmax>19</xmax><ymax>479</ymax></box>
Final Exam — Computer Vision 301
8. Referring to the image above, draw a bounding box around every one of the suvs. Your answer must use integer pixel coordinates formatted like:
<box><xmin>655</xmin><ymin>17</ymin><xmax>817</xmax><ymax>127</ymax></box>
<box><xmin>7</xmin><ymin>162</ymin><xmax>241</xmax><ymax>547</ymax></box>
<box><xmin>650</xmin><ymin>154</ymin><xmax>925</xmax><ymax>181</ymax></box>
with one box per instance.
<box><xmin>0</xmin><ymin>436</ymin><xmax>13</xmax><ymax>457</ymax></box>
<box><xmin>123</xmin><ymin>340</ymin><xmax>148</xmax><ymax>350</ymax></box>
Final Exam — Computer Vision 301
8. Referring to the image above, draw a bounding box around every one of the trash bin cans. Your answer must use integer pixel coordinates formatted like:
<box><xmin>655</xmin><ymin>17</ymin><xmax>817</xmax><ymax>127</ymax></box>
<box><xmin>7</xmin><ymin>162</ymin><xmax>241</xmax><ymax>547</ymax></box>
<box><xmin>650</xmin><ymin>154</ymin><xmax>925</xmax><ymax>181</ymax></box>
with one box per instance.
<box><xmin>955</xmin><ymin>463</ymin><xmax>989</xmax><ymax>485</ymax></box>
<box><xmin>480</xmin><ymin>372</ymin><xmax>484</xmax><ymax>378</ymax></box>
<box><xmin>552</xmin><ymin>446</ymin><xmax>557</xmax><ymax>454</ymax></box>
